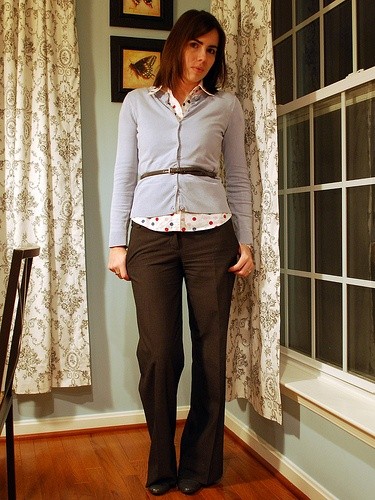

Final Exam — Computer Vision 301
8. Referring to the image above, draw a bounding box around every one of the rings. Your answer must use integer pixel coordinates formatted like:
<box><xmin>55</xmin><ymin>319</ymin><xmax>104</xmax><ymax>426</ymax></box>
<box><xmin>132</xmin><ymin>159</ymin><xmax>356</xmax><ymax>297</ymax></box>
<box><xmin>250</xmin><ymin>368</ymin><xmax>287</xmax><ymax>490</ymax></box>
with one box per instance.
<box><xmin>247</xmin><ymin>269</ymin><xmax>251</xmax><ymax>273</ymax></box>
<box><xmin>116</xmin><ymin>273</ymin><xmax>119</xmax><ymax>275</ymax></box>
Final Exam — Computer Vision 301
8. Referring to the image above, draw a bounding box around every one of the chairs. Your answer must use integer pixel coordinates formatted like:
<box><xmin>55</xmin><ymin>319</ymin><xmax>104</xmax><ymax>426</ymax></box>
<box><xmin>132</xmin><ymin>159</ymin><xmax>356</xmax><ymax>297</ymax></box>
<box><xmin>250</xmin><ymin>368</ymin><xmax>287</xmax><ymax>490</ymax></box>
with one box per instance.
<box><xmin>0</xmin><ymin>248</ymin><xmax>41</xmax><ymax>500</ymax></box>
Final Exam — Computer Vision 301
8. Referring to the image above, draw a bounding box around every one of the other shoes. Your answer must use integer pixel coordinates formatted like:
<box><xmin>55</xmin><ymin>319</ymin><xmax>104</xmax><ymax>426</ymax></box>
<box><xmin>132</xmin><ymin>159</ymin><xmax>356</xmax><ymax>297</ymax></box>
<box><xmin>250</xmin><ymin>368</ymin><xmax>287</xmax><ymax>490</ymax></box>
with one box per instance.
<box><xmin>149</xmin><ymin>484</ymin><xmax>174</xmax><ymax>496</ymax></box>
<box><xmin>180</xmin><ymin>482</ymin><xmax>202</xmax><ymax>494</ymax></box>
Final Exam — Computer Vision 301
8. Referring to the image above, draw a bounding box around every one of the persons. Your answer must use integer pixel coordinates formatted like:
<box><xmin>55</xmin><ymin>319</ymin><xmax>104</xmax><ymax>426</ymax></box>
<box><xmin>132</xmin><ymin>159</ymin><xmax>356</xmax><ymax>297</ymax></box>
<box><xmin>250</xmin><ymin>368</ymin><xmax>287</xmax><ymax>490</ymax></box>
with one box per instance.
<box><xmin>108</xmin><ymin>10</ymin><xmax>254</xmax><ymax>495</ymax></box>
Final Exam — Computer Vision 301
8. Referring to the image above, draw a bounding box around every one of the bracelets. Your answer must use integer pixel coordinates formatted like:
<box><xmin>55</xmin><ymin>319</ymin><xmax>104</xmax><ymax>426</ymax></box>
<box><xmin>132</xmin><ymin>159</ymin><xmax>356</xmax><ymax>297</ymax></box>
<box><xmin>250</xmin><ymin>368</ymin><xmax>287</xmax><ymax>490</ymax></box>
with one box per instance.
<box><xmin>246</xmin><ymin>245</ymin><xmax>254</xmax><ymax>253</ymax></box>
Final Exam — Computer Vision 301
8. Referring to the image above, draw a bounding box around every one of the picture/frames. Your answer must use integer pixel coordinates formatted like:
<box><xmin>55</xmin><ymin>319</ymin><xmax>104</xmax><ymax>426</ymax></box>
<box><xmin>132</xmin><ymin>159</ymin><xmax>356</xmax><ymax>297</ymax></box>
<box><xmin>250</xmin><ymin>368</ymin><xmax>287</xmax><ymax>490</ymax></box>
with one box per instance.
<box><xmin>108</xmin><ymin>0</ymin><xmax>174</xmax><ymax>31</ymax></box>
<box><xmin>109</xmin><ymin>35</ymin><xmax>166</xmax><ymax>102</ymax></box>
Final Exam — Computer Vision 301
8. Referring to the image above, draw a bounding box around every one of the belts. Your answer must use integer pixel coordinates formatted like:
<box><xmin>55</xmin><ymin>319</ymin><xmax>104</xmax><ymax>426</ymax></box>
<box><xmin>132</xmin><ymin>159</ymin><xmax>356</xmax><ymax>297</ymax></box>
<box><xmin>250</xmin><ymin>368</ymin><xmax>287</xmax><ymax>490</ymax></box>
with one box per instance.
<box><xmin>140</xmin><ymin>167</ymin><xmax>218</xmax><ymax>179</ymax></box>
